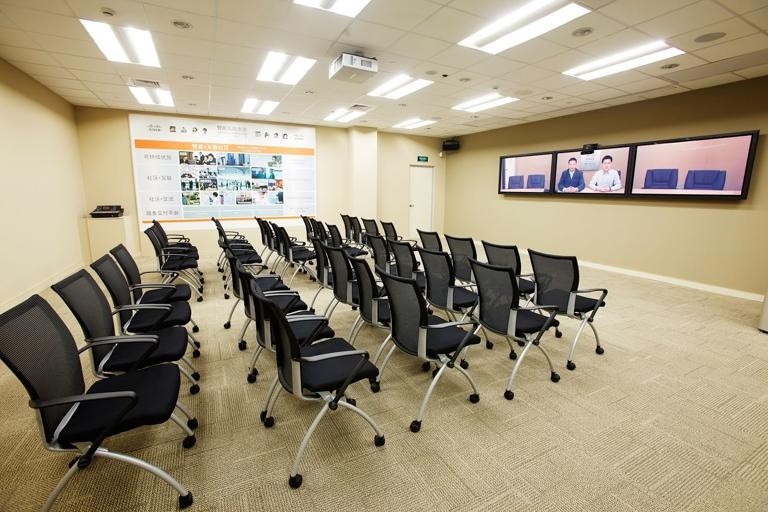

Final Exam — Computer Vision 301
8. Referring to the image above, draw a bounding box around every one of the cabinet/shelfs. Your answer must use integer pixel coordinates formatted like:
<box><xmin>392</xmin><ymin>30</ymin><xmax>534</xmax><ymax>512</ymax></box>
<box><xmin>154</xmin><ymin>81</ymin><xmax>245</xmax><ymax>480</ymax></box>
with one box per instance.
<box><xmin>85</xmin><ymin>216</ymin><xmax>137</xmax><ymax>265</ymax></box>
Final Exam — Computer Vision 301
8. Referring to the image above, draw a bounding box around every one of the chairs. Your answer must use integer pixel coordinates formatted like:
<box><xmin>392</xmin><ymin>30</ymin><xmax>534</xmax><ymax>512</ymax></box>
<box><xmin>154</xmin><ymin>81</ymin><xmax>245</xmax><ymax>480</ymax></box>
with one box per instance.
<box><xmin>301</xmin><ymin>215</ymin><xmax>348</xmax><ymax>246</ymax></box>
<box><xmin>345</xmin><ymin>254</ymin><xmax>407</xmax><ymax>348</ymax></box>
<box><xmin>443</xmin><ymin>234</ymin><xmax>486</xmax><ymax>295</ymax></box>
<box><xmin>232</xmin><ymin>231</ymin><xmax>261</xmax><ymax>263</ymax></box>
<box><xmin>311</xmin><ymin>237</ymin><xmax>331</xmax><ymax>312</ymax></box>
<box><xmin>150</xmin><ymin>225</ymin><xmax>204</xmax><ymax>285</ymax></box>
<box><xmin>464</xmin><ymin>256</ymin><xmax>561</xmax><ymax>401</ymax></box>
<box><xmin>143</xmin><ymin>228</ymin><xmax>207</xmax><ymax>292</ymax></box>
<box><xmin>86</xmin><ymin>338</ymin><xmax>197</xmax><ymax>508</ymax></box>
<box><xmin>110</xmin><ymin>243</ymin><xmax>200</xmax><ymax>333</ymax></box>
<box><xmin>247</xmin><ymin>278</ymin><xmax>335</xmax><ymax>383</ymax></box>
<box><xmin>341</xmin><ymin>213</ymin><xmax>406</xmax><ymax>248</ymax></box>
<box><xmin>261</xmin><ymin>245</ymin><xmax>305</xmax><ymax>287</ymax></box>
<box><xmin>417</xmin><ymin>245</ymin><xmax>480</xmax><ymax>331</ymax></box>
<box><xmin>90</xmin><ymin>254</ymin><xmax>203</xmax><ymax>357</ymax></box>
<box><xmin>86</xmin><ymin>270</ymin><xmax>200</xmax><ymax>394</ymax></box>
<box><xmin>480</xmin><ymin>240</ymin><xmax>545</xmax><ymax>317</ymax></box>
<box><xmin>524</xmin><ymin>247</ymin><xmax>608</xmax><ymax>370</ymax></box>
<box><xmin>224</xmin><ymin>259</ymin><xmax>276</xmax><ymax>329</ymax></box>
<box><xmin>255</xmin><ymin>218</ymin><xmax>316</xmax><ymax>260</ymax></box>
<box><xmin>416</xmin><ymin>228</ymin><xmax>452</xmax><ymax>263</ymax></box>
<box><xmin>322</xmin><ymin>244</ymin><xmax>385</xmax><ymax>325</ymax></box>
<box><xmin>234</xmin><ymin>260</ymin><xmax>298</xmax><ymax>351</ymax></box>
<box><xmin>370</xmin><ymin>265</ymin><xmax>482</xmax><ymax>433</ymax></box>
<box><xmin>250</xmin><ymin>279</ymin><xmax>386</xmax><ymax>489</ymax></box>
<box><xmin>210</xmin><ymin>215</ymin><xmax>232</xmax><ymax>297</ymax></box>
<box><xmin>390</xmin><ymin>240</ymin><xmax>417</xmax><ymax>282</ymax></box>
<box><xmin>154</xmin><ymin>221</ymin><xmax>190</xmax><ymax>243</ymax></box>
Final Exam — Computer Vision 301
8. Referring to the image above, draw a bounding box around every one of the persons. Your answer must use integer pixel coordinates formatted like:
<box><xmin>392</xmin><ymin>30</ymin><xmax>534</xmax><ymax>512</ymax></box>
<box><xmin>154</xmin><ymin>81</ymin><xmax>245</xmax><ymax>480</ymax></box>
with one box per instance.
<box><xmin>589</xmin><ymin>156</ymin><xmax>623</xmax><ymax>192</ymax></box>
<box><xmin>558</xmin><ymin>158</ymin><xmax>586</xmax><ymax>192</ymax></box>
<box><xmin>211</xmin><ymin>192</ymin><xmax>220</xmax><ymax>205</ymax></box>
<box><xmin>180</xmin><ymin>152</ymin><xmax>216</xmax><ymax>166</ymax></box>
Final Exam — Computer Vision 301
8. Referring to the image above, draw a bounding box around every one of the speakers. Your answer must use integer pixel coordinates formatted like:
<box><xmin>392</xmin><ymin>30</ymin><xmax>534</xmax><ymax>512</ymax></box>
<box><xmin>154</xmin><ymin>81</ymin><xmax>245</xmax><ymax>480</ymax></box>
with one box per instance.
<box><xmin>442</xmin><ymin>139</ymin><xmax>460</xmax><ymax>151</ymax></box>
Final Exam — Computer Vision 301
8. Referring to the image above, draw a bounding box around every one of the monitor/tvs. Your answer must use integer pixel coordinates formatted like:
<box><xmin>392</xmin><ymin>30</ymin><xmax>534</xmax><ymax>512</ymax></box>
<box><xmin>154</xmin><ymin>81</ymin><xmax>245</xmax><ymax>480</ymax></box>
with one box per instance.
<box><xmin>552</xmin><ymin>141</ymin><xmax>634</xmax><ymax>198</ymax></box>
<box><xmin>628</xmin><ymin>129</ymin><xmax>760</xmax><ymax>200</ymax></box>
<box><xmin>498</xmin><ymin>151</ymin><xmax>555</xmax><ymax>195</ymax></box>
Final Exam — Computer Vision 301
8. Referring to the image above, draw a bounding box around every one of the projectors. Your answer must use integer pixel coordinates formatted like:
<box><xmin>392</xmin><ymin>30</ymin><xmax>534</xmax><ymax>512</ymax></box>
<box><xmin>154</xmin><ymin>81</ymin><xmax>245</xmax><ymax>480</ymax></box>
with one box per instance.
<box><xmin>327</xmin><ymin>52</ymin><xmax>379</xmax><ymax>85</ymax></box>
